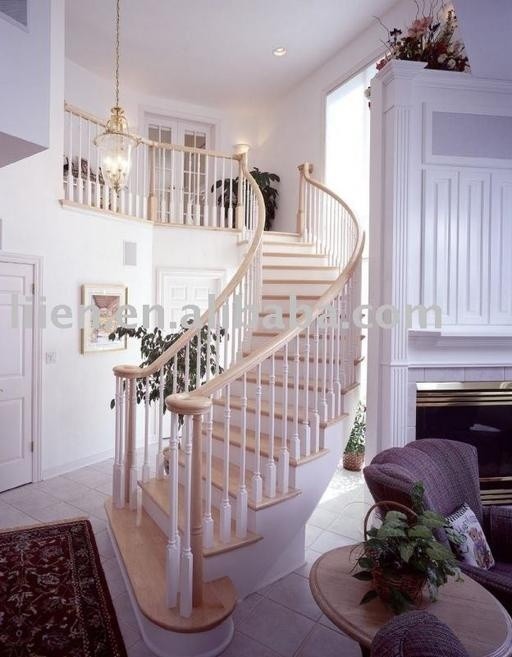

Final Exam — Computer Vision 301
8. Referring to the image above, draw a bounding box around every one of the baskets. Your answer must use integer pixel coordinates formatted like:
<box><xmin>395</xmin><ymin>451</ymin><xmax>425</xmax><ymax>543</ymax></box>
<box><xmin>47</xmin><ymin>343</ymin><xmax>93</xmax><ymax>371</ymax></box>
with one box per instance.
<box><xmin>361</xmin><ymin>500</ymin><xmax>427</xmax><ymax>608</ymax></box>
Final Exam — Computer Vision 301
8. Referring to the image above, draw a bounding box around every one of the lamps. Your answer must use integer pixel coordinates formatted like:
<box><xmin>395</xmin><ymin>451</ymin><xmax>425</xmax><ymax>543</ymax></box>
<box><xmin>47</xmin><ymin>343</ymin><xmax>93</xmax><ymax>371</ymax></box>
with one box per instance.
<box><xmin>93</xmin><ymin>2</ymin><xmax>141</xmax><ymax>202</ymax></box>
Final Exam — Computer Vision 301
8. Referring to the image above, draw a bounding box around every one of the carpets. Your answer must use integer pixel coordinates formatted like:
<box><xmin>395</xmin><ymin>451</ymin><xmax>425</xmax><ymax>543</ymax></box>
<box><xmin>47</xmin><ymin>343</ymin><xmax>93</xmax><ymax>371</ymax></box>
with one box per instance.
<box><xmin>0</xmin><ymin>515</ymin><xmax>130</xmax><ymax>655</ymax></box>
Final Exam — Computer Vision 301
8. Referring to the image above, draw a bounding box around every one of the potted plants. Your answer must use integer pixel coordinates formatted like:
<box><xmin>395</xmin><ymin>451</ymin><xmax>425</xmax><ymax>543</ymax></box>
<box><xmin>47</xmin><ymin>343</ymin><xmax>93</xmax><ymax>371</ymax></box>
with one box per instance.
<box><xmin>350</xmin><ymin>495</ymin><xmax>473</xmax><ymax>616</ymax></box>
<box><xmin>109</xmin><ymin>317</ymin><xmax>231</xmax><ymax>472</ymax></box>
<box><xmin>212</xmin><ymin>163</ymin><xmax>280</xmax><ymax>232</ymax></box>
<box><xmin>341</xmin><ymin>400</ymin><xmax>368</xmax><ymax>473</ymax></box>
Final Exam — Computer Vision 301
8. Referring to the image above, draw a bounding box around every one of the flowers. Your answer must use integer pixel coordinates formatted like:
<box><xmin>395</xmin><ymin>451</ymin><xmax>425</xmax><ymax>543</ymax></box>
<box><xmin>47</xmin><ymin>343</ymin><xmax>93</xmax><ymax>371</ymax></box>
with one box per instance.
<box><xmin>370</xmin><ymin>0</ymin><xmax>472</xmax><ymax>75</ymax></box>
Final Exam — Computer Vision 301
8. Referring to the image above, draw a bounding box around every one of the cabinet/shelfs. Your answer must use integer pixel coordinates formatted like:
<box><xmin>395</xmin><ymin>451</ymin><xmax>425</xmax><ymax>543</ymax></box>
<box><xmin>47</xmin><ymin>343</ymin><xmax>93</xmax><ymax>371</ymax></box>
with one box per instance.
<box><xmin>408</xmin><ymin>153</ymin><xmax>512</xmax><ymax>343</ymax></box>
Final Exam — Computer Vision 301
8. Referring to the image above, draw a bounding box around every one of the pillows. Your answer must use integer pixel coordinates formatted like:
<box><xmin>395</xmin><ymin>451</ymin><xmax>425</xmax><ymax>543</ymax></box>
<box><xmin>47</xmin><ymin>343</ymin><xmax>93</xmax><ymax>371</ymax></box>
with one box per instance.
<box><xmin>443</xmin><ymin>502</ymin><xmax>497</xmax><ymax>572</ymax></box>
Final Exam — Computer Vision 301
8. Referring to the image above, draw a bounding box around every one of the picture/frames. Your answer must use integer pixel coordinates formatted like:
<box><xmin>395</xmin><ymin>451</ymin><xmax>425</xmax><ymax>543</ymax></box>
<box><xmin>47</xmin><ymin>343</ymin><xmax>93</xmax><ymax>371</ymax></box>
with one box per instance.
<box><xmin>78</xmin><ymin>280</ymin><xmax>128</xmax><ymax>354</ymax></box>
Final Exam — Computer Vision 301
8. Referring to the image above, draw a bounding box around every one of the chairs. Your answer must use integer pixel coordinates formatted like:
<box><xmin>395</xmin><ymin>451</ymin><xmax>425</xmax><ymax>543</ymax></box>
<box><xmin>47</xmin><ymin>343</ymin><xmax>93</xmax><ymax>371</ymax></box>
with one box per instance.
<box><xmin>362</xmin><ymin>429</ymin><xmax>511</xmax><ymax>621</ymax></box>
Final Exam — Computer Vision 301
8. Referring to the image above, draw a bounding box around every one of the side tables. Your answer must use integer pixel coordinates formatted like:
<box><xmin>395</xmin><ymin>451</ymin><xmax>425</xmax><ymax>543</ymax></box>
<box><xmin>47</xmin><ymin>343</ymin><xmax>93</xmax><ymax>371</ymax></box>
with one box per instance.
<box><xmin>308</xmin><ymin>543</ymin><xmax>512</xmax><ymax>657</ymax></box>
